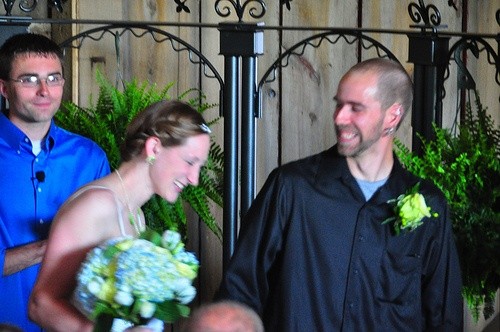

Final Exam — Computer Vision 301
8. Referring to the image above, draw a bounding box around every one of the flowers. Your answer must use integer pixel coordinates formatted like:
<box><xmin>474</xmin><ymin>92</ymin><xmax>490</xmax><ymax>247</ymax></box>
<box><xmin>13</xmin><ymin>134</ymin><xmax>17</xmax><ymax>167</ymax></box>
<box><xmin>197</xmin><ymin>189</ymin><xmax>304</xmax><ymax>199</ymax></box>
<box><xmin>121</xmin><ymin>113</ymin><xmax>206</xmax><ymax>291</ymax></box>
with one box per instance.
<box><xmin>380</xmin><ymin>181</ymin><xmax>439</xmax><ymax>235</ymax></box>
<box><xmin>75</xmin><ymin>228</ymin><xmax>201</xmax><ymax>332</ymax></box>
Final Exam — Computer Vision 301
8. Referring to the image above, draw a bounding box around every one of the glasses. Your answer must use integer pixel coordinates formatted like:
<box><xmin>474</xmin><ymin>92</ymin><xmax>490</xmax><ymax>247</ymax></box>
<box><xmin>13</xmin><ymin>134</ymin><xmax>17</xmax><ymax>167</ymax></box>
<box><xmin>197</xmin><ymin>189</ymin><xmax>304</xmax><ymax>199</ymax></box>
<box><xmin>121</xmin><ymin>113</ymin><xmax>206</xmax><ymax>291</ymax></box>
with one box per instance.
<box><xmin>9</xmin><ymin>75</ymin><xmax>65</xmax><ymax>88</ymax></box>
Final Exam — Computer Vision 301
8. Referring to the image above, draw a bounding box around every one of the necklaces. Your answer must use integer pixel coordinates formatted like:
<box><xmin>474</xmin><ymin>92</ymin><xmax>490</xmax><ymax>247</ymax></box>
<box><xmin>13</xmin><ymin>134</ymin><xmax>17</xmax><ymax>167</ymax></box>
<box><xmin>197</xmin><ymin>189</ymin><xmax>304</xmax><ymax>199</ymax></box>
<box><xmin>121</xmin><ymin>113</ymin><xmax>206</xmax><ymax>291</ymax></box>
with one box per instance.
<box><xmin>114</xmin><ymin>168</ymin><xmax>142</xmax><ymax>236</ymax></box>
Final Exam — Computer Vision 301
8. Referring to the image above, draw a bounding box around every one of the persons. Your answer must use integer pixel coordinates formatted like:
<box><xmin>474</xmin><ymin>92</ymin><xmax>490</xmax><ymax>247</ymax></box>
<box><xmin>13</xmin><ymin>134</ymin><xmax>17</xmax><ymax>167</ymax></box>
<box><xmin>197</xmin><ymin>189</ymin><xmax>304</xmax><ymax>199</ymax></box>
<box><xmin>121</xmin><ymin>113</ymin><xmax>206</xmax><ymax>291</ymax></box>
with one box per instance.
<box><xmin>27</xmin><ymin>100</ymin><xmax>212</xmax><ymax>332</ymax></box>
<box><xmin>212</xmin><ymin>57</ymin><xmax>466</xmax><ymax>332</ymax></box>
<box><xmin>0</xmin><ymin>32</ymin><xmax>113</xmax><ymax>332</ymax></box>
<box><xmin>187</xmin><ymin>299</ymin><xmax>265</xmax><ymax>332</ymax></box>
